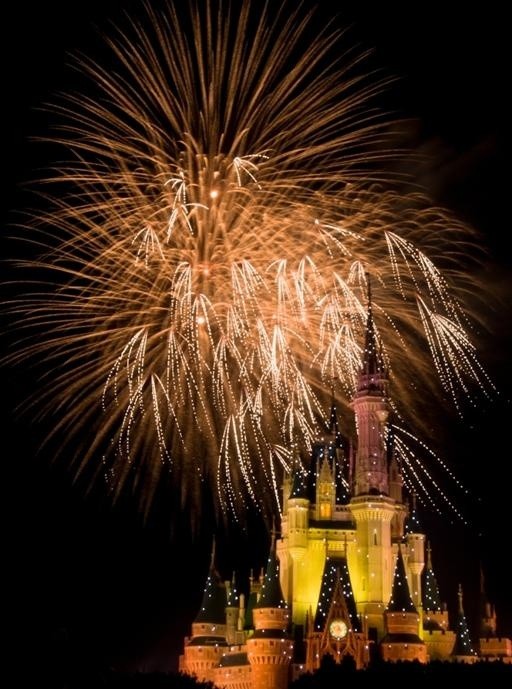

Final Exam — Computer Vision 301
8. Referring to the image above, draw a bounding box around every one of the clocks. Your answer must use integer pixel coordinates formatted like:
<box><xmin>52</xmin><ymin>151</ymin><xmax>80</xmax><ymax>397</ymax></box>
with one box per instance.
<box><xmin>329</xmin><ymin>620</ymin><xmax>347</xmax><ymax>638</ymax></box>
